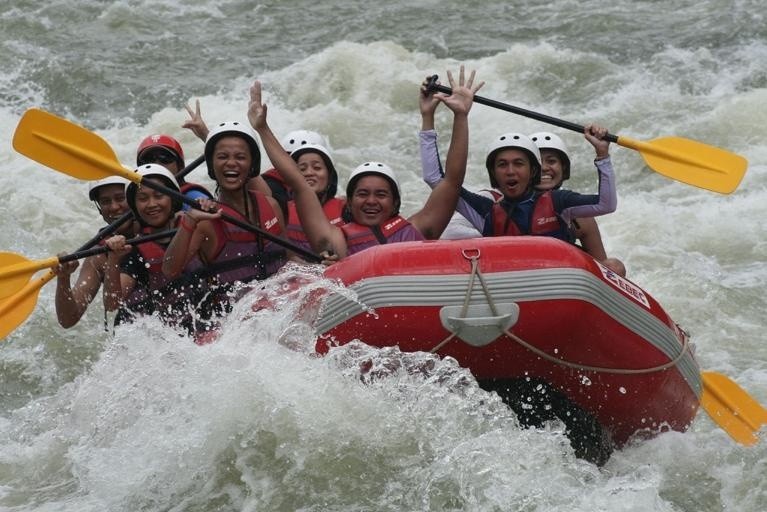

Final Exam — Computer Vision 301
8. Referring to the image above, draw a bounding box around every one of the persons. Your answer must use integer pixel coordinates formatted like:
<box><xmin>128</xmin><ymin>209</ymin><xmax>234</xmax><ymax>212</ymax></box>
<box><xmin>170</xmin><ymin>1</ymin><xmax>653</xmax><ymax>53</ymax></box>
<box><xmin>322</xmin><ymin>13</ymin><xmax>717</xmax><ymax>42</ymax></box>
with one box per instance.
<box><xmin>418</xmin><ymin>75</ymin><xmax>617</xmax><ymax>246</ymax></box>
<box><xmin>527</xmin><ymin>131</ymin><xmax>626</xmax><ymax>280</ymax></box>
<box><xmin>49</xmin><ymin>65</ymin><xmax>484</xmax><ymax>336</ymax></box>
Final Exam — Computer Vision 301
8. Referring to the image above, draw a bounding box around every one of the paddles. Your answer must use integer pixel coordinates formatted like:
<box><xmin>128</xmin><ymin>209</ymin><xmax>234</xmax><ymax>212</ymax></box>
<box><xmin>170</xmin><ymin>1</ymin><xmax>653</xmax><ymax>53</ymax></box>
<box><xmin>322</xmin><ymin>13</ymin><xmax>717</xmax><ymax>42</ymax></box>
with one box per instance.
<box><xmin>421</xmin><ymin>75</ymin><xmax>748</xmax><ymax>194</ymax></box>
<box><xmin>0</xmin><ymin>152</ymin><xmax>205</xmax><ymax>341</ymax></box>
<box><xmin>13</xmin><ymin>107</ymin><xmax>328</xmax><ymax>264</ymax></box>
<box><xmin>700</xmin><ymin>370</ymin><xmax>763</xmax><ymax>446</ymax></box>
<box><xmin>1</xmin><ymin>228</ymin><xmax>178</xmax><ymax>298</ymax></box>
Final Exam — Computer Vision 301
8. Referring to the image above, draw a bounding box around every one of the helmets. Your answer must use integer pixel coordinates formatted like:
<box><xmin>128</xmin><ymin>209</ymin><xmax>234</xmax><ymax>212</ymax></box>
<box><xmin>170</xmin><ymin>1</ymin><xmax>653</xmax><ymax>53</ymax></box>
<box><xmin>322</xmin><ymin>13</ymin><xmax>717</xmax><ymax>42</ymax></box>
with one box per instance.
<box><xmin>88</xmin><ymin>175</ymin><xmax>132</xmax><ymax>202</ymax></box>
<box><xmin>346</xmin><ymin>161</ymin><xmax>402</xmax><ymax>209</ymax></box>
<box><xmin>204</xmin><ymin>121</ymin><xmax>262</xmax><ymax>180</ymax></box>
<box><xmin>289</xmin><ymin>144</ymin><xmax>338</xmax><ymax>196</ymax></box>
<box><xmin>123</xmin><ymin>165</ymin><xmax>183</xmax><ymax>217</ymax></box>
<box><xmin>482</xmin><ymin>132</ymin><xmax>573</xmax><ymax>182</ymax></box>
<box><xmin>281</xmin><ymin>130</ymin><xmax>325</xmax><ymax>152</ymax></box>
<box><xmin>136</xmin><ymin>134</ymin><xmax>185</xmax><ymax>173</ymax></box>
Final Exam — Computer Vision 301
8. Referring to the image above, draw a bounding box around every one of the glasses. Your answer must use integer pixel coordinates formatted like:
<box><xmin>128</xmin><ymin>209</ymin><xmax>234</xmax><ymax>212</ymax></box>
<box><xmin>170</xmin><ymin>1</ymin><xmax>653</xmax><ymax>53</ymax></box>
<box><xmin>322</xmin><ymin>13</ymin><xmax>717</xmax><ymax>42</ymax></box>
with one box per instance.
<box><xmin>141</xmin><ymin>150</ymin><xmax>174</xmax><ymax>164</ymax></box>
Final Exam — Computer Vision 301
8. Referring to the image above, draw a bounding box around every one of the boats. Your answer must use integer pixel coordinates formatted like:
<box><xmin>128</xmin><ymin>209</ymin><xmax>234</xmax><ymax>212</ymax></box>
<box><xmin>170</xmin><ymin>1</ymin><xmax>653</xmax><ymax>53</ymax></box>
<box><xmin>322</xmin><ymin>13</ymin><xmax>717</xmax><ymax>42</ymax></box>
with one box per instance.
<box><xmin>185</xmin><ymin>233</ymin><xmax>702</xmax><ymax>465</ymax></box>
